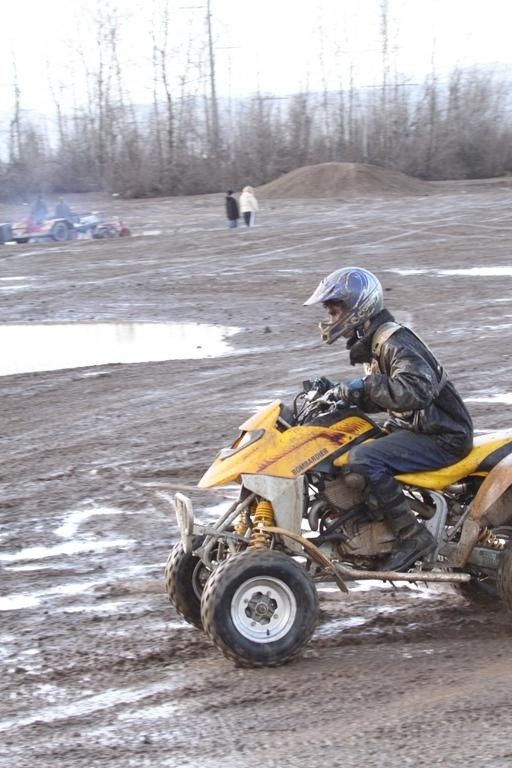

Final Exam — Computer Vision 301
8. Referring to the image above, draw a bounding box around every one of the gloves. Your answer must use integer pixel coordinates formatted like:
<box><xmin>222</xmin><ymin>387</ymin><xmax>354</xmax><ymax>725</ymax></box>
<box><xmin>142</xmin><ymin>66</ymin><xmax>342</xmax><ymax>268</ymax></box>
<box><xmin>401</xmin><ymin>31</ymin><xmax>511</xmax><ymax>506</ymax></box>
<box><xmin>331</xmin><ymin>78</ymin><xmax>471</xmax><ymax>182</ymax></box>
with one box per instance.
<box><xmin>315</xmin><ymin>376</ymin><xmax>364</xmax><ymax>412</ymax></box>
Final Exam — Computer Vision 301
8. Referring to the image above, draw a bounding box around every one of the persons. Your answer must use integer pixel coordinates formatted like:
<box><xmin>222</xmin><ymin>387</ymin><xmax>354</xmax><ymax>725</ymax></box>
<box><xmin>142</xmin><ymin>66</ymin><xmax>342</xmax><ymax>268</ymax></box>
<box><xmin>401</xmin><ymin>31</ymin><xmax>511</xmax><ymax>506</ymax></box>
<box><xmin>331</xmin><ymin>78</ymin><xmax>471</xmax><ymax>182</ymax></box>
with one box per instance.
<box><xmin>30</xmin><ymin>196</ymin><xmax>47</xmax><ymax>224</ymax></box>
<box><xmin>310</xmin><ymin>268</ymin><xmax>472</xmax><ymax>572</ymax></box>
<box><xmin>55</xmin><ymin>197</ymin><xmax>71</xmax><ymax>222</ymax></box>
<box><xmin>239</xmin><ymin>186</ymin><xmax>258</xmax><ymax>229</ymax></box>
<box><xmin>226</xmin><ymin>190</ymin><xmax>239</xmax><ymax>227</ymax></box>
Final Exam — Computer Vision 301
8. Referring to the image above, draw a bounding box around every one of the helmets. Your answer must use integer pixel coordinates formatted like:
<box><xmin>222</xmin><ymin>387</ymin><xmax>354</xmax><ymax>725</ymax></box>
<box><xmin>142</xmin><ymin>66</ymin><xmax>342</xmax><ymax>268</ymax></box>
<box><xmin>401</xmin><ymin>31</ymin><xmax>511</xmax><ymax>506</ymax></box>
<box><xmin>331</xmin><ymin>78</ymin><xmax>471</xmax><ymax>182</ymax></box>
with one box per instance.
<box><xmin>301</xmin><ymin>266</ymin><xmax>384</xmax><ymax>344</ymax></box>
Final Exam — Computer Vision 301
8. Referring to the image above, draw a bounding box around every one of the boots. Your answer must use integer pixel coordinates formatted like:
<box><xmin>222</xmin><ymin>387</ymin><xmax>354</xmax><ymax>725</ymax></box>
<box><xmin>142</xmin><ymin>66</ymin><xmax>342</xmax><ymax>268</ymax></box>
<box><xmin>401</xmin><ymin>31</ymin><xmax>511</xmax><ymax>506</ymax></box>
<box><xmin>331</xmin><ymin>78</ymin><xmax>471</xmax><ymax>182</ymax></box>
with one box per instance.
<box><xmin>375</xmin><ymin>484</ymin><xmax>437</xmax><ymax>571</ymax></box>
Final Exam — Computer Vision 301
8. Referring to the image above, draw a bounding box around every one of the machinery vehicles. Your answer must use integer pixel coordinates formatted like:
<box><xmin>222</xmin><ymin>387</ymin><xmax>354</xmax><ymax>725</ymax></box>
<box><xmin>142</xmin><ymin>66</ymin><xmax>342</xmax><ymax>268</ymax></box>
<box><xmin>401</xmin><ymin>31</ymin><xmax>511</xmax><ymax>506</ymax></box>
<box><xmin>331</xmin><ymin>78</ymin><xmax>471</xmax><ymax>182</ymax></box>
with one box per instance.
<box><xmin>1</xmin><ymin>210</ymin><xmax>129</xmax><ymax>243</ymax></box>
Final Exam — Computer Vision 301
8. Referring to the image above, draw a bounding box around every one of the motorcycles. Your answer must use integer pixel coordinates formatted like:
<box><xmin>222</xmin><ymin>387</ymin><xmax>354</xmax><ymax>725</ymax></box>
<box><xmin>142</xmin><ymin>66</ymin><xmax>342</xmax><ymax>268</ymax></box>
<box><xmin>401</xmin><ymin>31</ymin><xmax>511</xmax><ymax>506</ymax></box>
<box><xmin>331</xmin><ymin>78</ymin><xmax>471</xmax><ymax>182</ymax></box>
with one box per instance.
<box><xmin>164</xmin><ymin>376</ymin><xmax>512</xmax><ymax>668</ymax></box>
